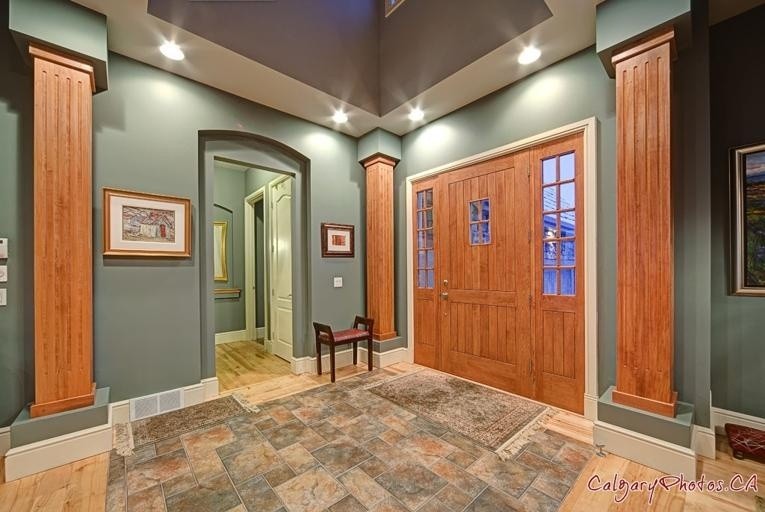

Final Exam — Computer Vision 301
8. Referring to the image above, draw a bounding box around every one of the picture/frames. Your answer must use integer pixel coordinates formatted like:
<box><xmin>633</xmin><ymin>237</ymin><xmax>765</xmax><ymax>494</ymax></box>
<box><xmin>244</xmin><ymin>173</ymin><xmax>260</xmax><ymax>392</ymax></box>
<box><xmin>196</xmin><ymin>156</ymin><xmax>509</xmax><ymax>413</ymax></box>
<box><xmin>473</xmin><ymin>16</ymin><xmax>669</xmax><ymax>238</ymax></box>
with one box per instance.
<box><xmin>102</xmin><ymin>185</ymin><xmax>192</xmax><ymax>260</ymax></box>
<box><xmin>728</xmin><ymin>141</ymin><xmax>765</xmax><ymax>296</ymax></box>
<box><xmin>320</xmin><ymin>223</ymin><xmax>355</xmax><ymax>258</ymax></box>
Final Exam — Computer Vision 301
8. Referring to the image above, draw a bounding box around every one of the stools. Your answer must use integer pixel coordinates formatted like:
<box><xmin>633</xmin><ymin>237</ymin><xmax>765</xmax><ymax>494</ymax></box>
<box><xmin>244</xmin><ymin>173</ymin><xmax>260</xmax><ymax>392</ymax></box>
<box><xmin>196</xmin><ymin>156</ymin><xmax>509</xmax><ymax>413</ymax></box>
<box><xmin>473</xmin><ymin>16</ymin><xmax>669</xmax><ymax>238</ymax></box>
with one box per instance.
<box><xmin>313</xmin><ymin>315</ymin><xmax>375</xmax><ymax>382</ymax></box>
